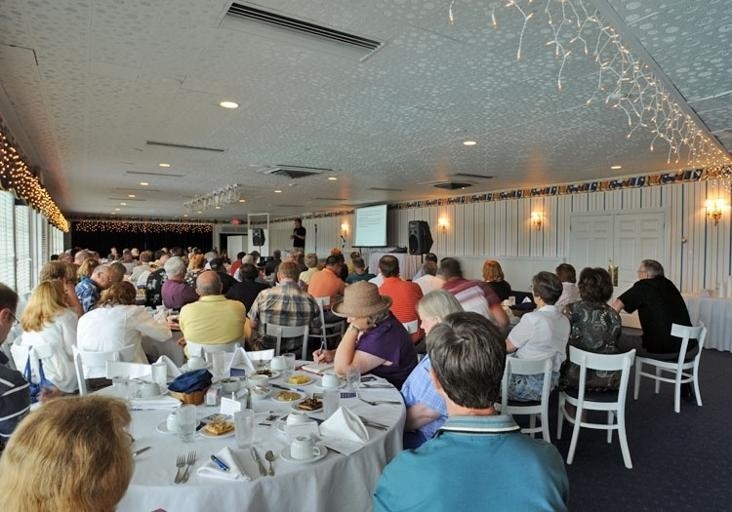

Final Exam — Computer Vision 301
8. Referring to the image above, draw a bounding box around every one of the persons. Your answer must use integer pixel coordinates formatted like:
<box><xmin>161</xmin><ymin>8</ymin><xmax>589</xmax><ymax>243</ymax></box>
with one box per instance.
<box><xmin>1</xmin><ymin>283</ymin><xmax>31</xmax><ymax>457</ymax></box>
<box><xmin>108</xmin><ymin>247</ymin><xmax>376</xmax><ymax>362</ymax></box>
<box><xmin>0</xmin><ymin>245</ymin><xmax>622</xmax><ymax>512</ymax></box>
<box><xmin>368</xmin><ymin>253</ymin><xmax>513</xmax><ymax>360</ymax></box>
<box><xmin>398</xmin><ymin>289</ymin><xmax>464</xmax><ymax>450</ymax></box>
<box><xmin>288</xmin><ymin>218</ymin><xmax>306</xmax><ymax>252</ymax></box>
<box><xmin>10</xmin><ymin>247</ymin><xmax>173</xmax><ymax>395</ymax></box>
<box><xmin>312</xmin><ymin>280</ymin><xmax>419</xmax><ymax>390</ymax></box>
<box><xmin>498</xmin><ymin>263</ymin><xmax>621</xmax><ymax>431</ymax></box>
<box><xmin>611</xmin><ymin>259</ymin><xmax>699</xmax><ymax>400</ymax></box>
<box><xmin>1</xmin><ymin>395</ymin><xmax>134</xmax><ymax>510</ymax></box>
<box><xmin>371</xmin><ymin>311</ymin><xmax>570</xmax><ymax>512</ymax></box>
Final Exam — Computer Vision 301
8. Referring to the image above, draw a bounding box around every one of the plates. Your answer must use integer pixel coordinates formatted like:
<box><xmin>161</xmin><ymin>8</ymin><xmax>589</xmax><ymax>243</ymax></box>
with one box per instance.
<box><xmin>120</xmin><ymin>351</ymin><xmax>402</xmax><ymax>485</ymax></box>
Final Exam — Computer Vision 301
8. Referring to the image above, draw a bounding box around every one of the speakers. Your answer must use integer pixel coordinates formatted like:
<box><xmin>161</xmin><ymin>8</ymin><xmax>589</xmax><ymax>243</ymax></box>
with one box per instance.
<box><xmin>252</xmin><ymin>228</ymin><xmax>265</xmax><ymax>246</ymax></box>
<box><xmin>409</xmin><ymin>220</ymin><xmax>433</xmax><ymax>255</ymax></box>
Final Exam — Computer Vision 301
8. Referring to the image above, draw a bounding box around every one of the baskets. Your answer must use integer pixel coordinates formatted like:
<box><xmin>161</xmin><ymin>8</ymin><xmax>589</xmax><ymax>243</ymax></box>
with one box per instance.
<box><xmin>167</xmin><ymin>385</ymin><xmax>209</xmax><ymax>405</ymax></box>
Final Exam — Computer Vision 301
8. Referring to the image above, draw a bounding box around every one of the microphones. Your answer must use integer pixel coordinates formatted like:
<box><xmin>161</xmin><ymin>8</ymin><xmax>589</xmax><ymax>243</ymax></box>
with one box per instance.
<box><xmin>314</xmin><ymin>224</ymin><xmax>317</xmax><ymax>232</ymax></box>
<box><xmin>341</xmin><ymin>235</ymin><xmax>346</xmax><ymax>242</ymax></box>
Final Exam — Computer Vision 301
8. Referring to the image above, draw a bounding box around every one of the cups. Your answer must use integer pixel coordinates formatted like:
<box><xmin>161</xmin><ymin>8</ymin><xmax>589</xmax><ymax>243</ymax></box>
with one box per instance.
<box><xmin>145</xmin><ymin>305</ymin><xmax>179</xmax><ymax>326</ymax></box>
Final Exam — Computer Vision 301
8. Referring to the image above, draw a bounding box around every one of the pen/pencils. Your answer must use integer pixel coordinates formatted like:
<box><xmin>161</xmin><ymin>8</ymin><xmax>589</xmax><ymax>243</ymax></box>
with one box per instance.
<box><xmin>210</xmin><ymin>454</ymin><xmax>229</xmax><ymax>471</ymax></box>
<box><xmin>316</xmin><ymin>342</ymin><xmax>324</xmax><ymax>367</ymax></box>
<box><xmin>270</xmin><ymin>383</ymin><xmax>305</xmax><ymax>393</ymax></box>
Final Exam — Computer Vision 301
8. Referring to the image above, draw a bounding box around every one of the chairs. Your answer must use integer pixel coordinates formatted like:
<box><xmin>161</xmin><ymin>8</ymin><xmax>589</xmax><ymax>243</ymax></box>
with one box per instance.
<box><xmin>10</xmin><ymin>296</ymin><xmax>707</xmax><ymax>469</ymax></box>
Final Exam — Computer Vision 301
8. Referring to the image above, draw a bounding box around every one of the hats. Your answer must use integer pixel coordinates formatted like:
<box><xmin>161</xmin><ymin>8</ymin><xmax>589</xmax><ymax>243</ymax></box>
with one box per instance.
<box><xmin>331</xmin><ymin>280</ymin><xmax>392</xmax><ymax>317</ymax></box>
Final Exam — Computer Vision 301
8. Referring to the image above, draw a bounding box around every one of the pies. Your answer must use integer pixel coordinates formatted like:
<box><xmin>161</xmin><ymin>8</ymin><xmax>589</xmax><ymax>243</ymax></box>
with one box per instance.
<box><xmin>205</xmin><ymin>420</ymin><xmax>233</xmax><ymax>436</ymax></box>
